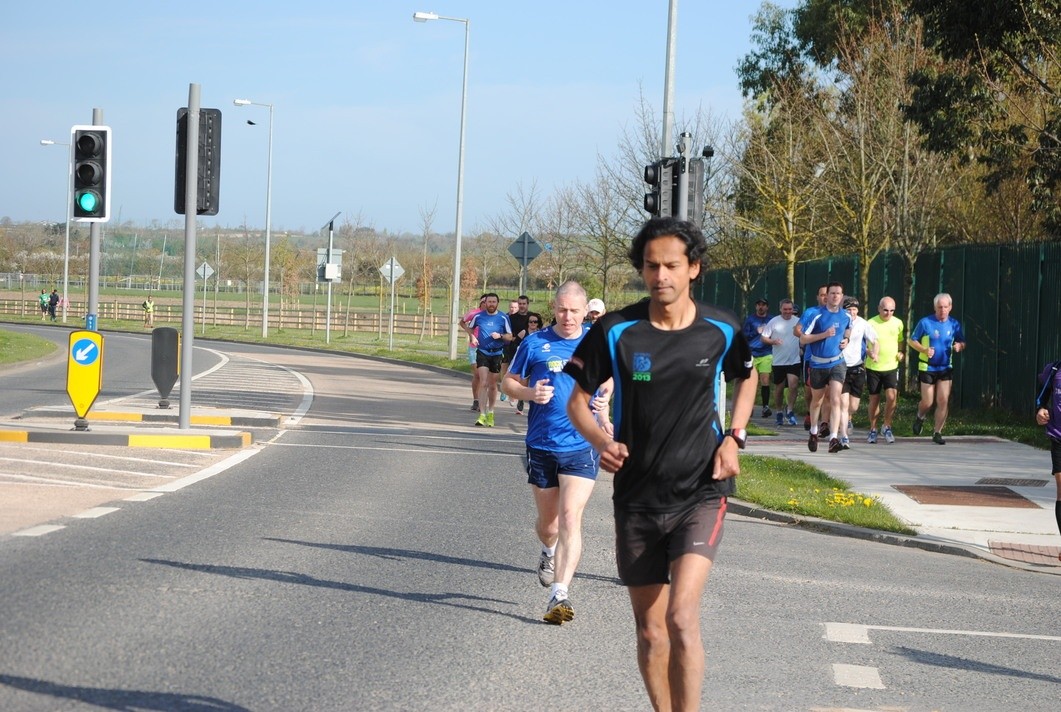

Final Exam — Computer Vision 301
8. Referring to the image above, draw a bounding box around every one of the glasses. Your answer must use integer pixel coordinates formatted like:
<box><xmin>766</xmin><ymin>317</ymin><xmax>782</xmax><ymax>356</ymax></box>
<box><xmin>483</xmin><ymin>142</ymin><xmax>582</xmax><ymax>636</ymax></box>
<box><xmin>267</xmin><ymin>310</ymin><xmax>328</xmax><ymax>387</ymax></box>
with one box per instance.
<box><xmin>792</xmin><ymin>313</ymin><xmax>799</xmax><ymax>315</ymax></box>
<box><xmin>880</xmin><ymin>308</ymin><xmax>896</xmax><ymax>312</ymax></box>
<box><xmin>528</xmin><ymin>320</ymin><xmax>540</xmax><ymax>323</ymax></box>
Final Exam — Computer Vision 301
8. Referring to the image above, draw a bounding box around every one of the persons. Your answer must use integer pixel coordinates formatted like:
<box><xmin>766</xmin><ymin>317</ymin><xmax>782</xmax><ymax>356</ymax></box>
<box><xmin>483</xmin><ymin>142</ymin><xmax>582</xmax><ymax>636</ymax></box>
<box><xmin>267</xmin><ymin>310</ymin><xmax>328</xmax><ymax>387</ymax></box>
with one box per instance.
<box><xmin>1036</xmin><ymin>358</ymin><xmax>1061</xmax><ymax>565</ymax></box>
<box><xmin>40</xmin><ymin>289</ymin><xmax>70</xmax><ymax>321</ymax></box>
<box><xmin>501</xmin><ymin>280</ymin><xmax>613</xmax><ymax>626</ymax></box>
<box><xmin>142</xmin><ymin>296</ymin><xmax>155</xmax><ymax>327</ymax></box>
<box><xmin>563</xmin><ymin>218</ymin><xmax>761</xmax><ymax>711</ymax></box>
<box><xmin>459</xmin><ymin>282</ymin><xmax>967</xmax><ymax>453</ymax></box>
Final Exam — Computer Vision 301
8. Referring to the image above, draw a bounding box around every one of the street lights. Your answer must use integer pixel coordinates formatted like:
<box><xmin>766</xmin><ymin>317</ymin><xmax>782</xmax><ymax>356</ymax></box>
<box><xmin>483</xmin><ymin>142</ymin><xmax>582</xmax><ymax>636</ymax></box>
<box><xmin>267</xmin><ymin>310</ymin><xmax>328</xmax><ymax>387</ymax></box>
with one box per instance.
<box><xmin>233</xmin><ymin>98</ymin><xmax>273</xmax><ymax>337</ymax></box>
<box><xmin>40</xmin><ymin>139</ymin><xmax>72</xmax><ymax>323</ymax></box>
<box><xmin>413</xmin><ymin>10</ymin><xmax>470</xmax><ymax>363</ymax></box>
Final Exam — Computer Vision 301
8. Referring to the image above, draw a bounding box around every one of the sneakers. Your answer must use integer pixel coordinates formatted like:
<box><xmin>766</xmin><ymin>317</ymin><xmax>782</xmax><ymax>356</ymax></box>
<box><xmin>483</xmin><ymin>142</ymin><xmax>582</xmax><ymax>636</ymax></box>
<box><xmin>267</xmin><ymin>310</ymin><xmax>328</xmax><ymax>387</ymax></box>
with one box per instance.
<box><xmin>751</xmin><ymin>405</ymin><xmax>946</xmax><ymax>453</ymax></box>
<box><xmin>471</xmin><ymin>393</ymin><xmax>524</xmax><ymax>427</ymax></box>
<box><xmin>543</xmin><ymin>589</ymin><xmax>574</xmax><ymax>625</ymax></box>
<box><xmin>538</xmin><ymin>547</ymin><xmax>556</xmax><ymax>587</ymax></box>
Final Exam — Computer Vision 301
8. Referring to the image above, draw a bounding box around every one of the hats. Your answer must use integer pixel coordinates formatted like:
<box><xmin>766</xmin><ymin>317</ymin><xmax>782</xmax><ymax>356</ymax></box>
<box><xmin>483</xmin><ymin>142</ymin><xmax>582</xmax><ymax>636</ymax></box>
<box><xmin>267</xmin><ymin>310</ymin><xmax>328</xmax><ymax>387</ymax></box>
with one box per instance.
<box><xmin>755</xmin><ymin>298</ymin><xmax>769</xmax><ymax>304</ymax></box>
<box><xmin>588</xmin><ymin>298</ymin><xmax>606</xmax><ymax>313</ymax></box>
<box><xmin>793</xmin><ymin>305</ymin><xmax>799</xmax><ymax>311</ymax></box>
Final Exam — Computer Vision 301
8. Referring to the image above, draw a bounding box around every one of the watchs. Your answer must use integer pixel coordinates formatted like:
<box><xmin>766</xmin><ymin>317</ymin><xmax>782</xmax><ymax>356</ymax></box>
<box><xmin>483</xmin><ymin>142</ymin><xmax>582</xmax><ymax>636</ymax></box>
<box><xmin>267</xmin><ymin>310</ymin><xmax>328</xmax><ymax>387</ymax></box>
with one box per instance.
<box><xmin>725</xmin><ymin>429</ymin><xmax>748</xmax><ymax>451</ymax></box>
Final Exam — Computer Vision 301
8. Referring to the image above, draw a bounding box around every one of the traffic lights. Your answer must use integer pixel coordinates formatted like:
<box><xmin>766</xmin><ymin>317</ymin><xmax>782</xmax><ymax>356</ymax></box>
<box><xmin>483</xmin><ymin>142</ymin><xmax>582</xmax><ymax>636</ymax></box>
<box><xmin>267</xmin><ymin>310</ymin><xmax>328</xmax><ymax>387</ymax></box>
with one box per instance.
<box><xmin>70</xmin><ymin>125</ymin><xmax>111</xmax><ymax>222</ymax></box>
<box><xmin>643</xmin><ymin>157</ymin><xmax>674</xmax><ymax>219</ymax></box>
